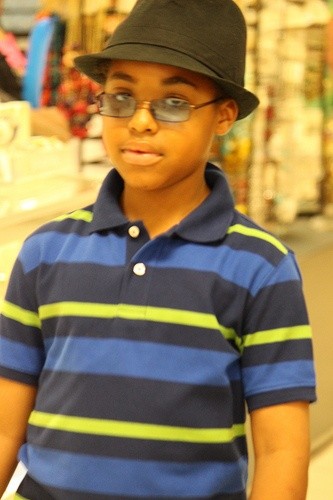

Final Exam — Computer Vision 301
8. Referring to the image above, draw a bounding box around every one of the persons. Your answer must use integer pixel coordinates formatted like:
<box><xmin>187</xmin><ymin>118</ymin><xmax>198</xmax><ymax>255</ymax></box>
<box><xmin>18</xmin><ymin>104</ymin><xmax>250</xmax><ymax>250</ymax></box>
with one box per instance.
<box><xmin>0</xmin><ymin>1</ymin><xmax>331</xmax><ymax>499</ymax></box>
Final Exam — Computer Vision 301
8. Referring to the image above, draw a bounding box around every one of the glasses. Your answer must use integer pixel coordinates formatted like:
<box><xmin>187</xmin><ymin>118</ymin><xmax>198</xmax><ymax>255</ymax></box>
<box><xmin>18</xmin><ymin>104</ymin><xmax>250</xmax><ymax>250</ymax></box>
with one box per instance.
<box><xmin>95</xmin><ymin>90</ymin><xmax>221</xmax><ymax>123</ymax></box>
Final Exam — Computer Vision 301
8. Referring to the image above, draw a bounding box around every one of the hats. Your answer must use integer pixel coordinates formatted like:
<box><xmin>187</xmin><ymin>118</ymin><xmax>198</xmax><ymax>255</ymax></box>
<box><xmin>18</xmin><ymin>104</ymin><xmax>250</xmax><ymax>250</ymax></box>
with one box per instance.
<box><xmin>73</xmin><ymin>0</ymin><xmax>260</xmax><ymax>122</ymax></box>
